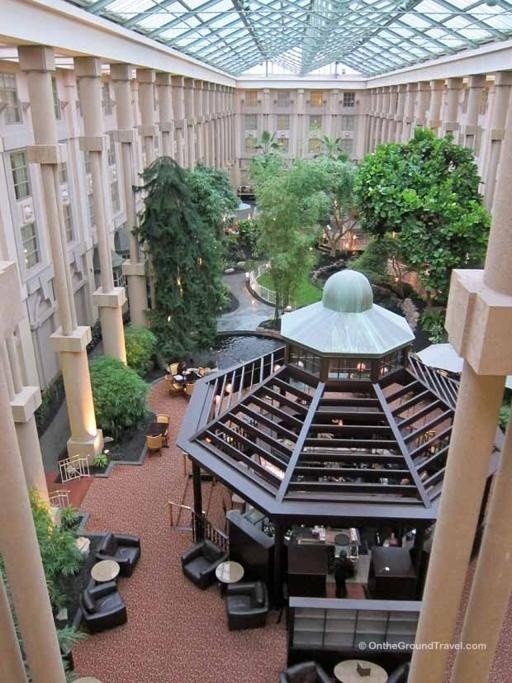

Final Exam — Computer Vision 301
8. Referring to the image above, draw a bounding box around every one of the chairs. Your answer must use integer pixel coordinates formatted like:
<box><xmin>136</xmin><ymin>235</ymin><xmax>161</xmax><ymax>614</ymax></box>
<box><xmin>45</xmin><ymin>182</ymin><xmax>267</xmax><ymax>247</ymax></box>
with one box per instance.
<box><xmin>77</xmin><ymin>580</ymin><xmax>131</xmax><ymax>635</ymax></box>
<box><xmin>386</xmin><ymin>659</ymin><xmax>411</xmax><ymax>683</ymax></box>
<box><xmin>224</xmin><ymin>579</ymin><xmax>271</xmax><ymax>631</ymax></box>
<box><xmin>93</xmin><ymin>530</ymin><xmax>143</xmax><ymax>579</ymax></box>
<box><xmin>276</xmin><ymin>657</ymin><xmax>333</xmax><ymax>682</ymax></box>
<box><xmin>145</xmin><ymin>433</ymin><xmax>163</xmax><ymax>457</ymax></box>
<box><xmin>166</xmin><ymin>361</ymin><xmax>217</xmax><ymax>400</ymax></box>
<box><xmin>154</xmin><ymin>414</ymin><xmax>171</xmax><ymax>448</ymax></box>
<box><xmin>179</xmin><ymin>537</ymin><xmax>230</xmax><ymax>590</ymax></box>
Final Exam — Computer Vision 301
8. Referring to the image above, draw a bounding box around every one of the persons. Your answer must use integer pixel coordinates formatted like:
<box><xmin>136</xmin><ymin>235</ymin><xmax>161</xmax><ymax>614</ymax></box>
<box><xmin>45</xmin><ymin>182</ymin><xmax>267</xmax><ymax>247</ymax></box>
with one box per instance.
<box><xmin>177</xmin><ymin>361</ymin><xmax>187</xmax><ymax>373</ymax></box>
<box><xmin>277</xmin><ymin>417</ymin><xmax>414</xmax><ymax>598</ymax></box>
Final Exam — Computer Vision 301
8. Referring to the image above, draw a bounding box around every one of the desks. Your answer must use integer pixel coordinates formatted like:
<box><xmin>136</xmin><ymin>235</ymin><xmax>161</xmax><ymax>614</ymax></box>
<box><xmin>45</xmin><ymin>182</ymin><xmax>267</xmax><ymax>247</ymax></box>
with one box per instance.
<box><xmin>89</xmin><ymin>558</ymin><xmax>121</xmax><ymax>585</ymax></box>
<box><xmin>214</xmin><ymin>559</ymin><xmax>246</xmax><ymax>599</ymax></box>
<box><xmin>146</xmin><ymin>422</ymin><xmax>170</xmax><ymax>436</ymax></box>
<box><xmin>330</xmin><ymin>658</ymin><xmax>390</xmax><ymax>683</ymax></box>
